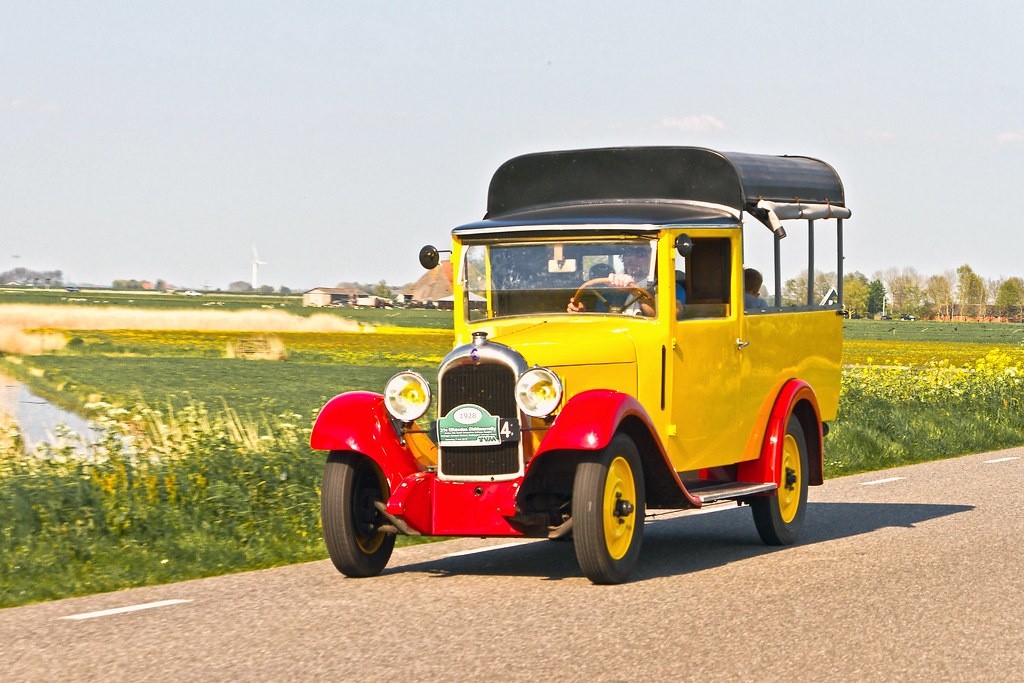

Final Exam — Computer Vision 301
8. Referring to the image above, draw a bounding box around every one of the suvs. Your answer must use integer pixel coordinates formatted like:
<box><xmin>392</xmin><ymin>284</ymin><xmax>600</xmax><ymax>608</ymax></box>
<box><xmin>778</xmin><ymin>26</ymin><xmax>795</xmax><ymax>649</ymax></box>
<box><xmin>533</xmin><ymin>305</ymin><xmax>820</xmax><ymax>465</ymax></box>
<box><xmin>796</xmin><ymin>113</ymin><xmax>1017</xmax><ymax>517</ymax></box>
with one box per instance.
<box><xmin>309</xmin><ymin>144</ymin><xmax>853</xmax><ymax>586</ymax></box>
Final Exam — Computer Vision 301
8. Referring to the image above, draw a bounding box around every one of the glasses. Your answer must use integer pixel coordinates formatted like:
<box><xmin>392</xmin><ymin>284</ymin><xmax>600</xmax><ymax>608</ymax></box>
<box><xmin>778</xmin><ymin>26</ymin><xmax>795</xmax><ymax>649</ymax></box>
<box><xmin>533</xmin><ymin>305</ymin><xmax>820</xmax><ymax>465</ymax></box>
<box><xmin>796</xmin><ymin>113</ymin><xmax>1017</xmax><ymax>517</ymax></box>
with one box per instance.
<box><xmin>623</xmin><ymin>247</ymin><xmax>647</xmax><ymax>257</ymax></box>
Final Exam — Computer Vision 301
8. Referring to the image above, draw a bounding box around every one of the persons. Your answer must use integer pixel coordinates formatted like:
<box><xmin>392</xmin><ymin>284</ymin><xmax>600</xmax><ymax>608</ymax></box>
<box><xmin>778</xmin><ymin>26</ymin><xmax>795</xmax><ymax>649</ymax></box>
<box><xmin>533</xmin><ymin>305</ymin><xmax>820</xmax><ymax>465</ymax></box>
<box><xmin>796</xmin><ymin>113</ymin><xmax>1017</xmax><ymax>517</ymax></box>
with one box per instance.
<box><xmin>744</xmin><ymin>268</ymin><xmax>769</xmax><ymax>309</ymax></box>
<box><xmin>567</xmin><ymin>246</ymin><xmax>685</xmax><ymax>320</ymax></box>
